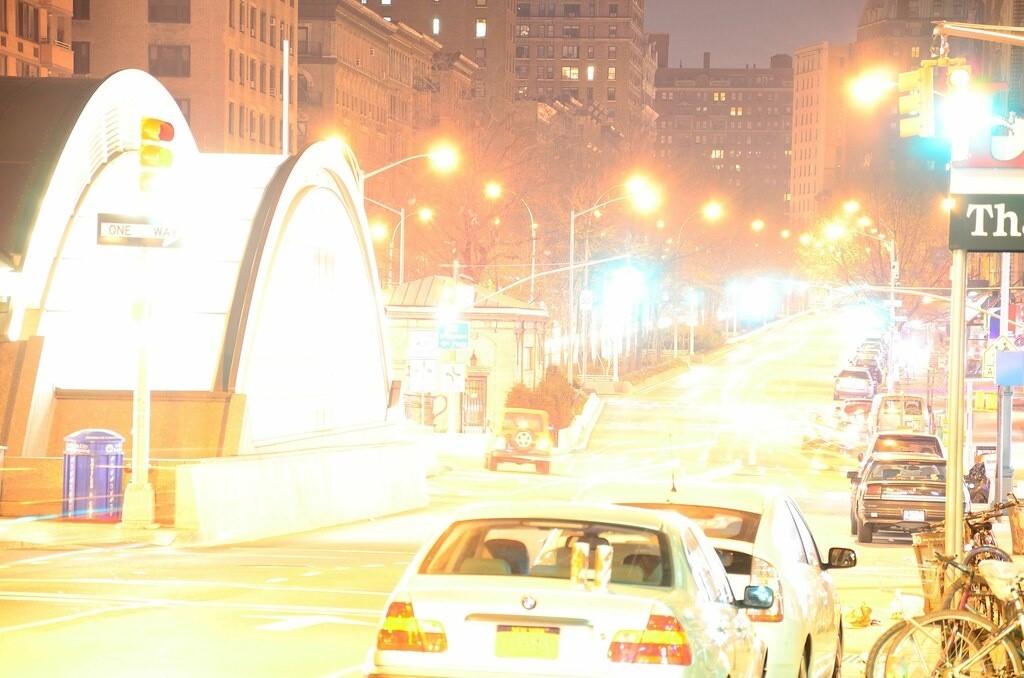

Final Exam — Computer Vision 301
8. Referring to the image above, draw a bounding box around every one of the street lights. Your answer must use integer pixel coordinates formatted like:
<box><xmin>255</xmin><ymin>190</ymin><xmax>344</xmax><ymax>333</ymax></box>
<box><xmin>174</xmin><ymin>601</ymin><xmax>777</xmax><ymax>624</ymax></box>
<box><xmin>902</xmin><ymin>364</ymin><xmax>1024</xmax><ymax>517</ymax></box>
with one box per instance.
<box><xmin>389</xmin><ymin>209</ymin><xmax>432</xmax><ymax>288</ymax></box>
<box><xmin>569</xmin><ymin>176</ymin><xmax>659</xmax><ymax>384</ymax></box>
<box><xmin>485</xmin><ymin>183</ymin><xmax>536</xmax><ymax>307</ymax></box>
<box><xmin>674</xmin><ymin>202</ymin><xmax>720</xmax><ymax>357</ymax></box>
<box><xmin>732</xmin><ymin>202</ymin><xmax>898</xmax><ymax>394</ymax></box>
<box><xmin>328</xmin><ymin>135</ymin><xmax>459</xmax><ymax>206</ymax></box>
<box><xmin>848</xmin><ymin>73</ymin><xmax>965</xmax><ymax>608</ymax></box>
<box><xmin>939</xmin><ymin>89</ymin><xmax>1018</xmax><ymax>523</ymax></box>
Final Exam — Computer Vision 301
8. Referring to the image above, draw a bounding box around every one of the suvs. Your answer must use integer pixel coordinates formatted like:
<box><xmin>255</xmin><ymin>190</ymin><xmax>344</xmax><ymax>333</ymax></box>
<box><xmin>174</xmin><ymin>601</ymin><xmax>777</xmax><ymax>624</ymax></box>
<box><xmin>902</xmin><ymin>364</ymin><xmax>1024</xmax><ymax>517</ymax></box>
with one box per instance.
<box><xmin>484</xmin><ymin>408</ymin><xmax>554</xmax><ymax>475</ymax></box>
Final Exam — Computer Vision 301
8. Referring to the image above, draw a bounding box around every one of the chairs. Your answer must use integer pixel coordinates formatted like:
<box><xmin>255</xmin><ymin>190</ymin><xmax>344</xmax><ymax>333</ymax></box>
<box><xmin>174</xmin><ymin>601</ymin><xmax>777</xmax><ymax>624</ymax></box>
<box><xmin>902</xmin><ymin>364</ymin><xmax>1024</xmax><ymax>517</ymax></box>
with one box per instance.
<box><xmin>624</xmin><ymin>553</ymin><xmax>661</xmax><ymax>579</ymax></box>
<box><xmin>921</xmin><ymin>467</ymin><xmax>936</xmax><ymax>479</ymax></box>
<box><xmin>486</xmin><ymin>539</ymin><xmax>529</xmax><ymax>574</ymax></box>
<box><xmin>884</xmin><ymin>468</ymin><xmax>901</xmax><ymax>479</ymax></box>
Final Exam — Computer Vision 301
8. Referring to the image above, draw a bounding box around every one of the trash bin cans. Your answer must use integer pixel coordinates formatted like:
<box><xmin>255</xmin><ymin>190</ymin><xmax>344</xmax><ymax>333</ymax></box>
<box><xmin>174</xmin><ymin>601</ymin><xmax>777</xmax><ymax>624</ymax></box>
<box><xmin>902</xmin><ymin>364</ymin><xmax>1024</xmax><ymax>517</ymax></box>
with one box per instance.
<box><xmin>463</xmin><ymin>365</ymin><xmax>490</xmax><ymax>426</ymax></box>
<box><xmin>62</xmin><ymin>429</ymin><xmax>126</xmax><ymax>518</ymax></box>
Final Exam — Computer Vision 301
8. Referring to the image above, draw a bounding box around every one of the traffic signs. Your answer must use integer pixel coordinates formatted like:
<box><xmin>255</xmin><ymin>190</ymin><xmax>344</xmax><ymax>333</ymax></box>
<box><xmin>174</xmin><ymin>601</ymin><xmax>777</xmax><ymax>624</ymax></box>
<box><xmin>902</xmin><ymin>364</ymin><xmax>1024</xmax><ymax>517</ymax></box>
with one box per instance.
<box><xmin>97</xmin><ymin>213</ymin><xmax>185</xmax><ymax>249</ymax></box>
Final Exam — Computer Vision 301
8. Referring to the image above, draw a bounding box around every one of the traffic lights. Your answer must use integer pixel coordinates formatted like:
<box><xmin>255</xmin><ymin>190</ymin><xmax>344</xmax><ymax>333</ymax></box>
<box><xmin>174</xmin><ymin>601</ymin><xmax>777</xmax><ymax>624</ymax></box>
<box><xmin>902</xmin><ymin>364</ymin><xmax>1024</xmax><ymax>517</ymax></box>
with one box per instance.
<box><xmin>139</xmin><ymin>118</ymin><xmax>174</xmax><ymax>200</ymax></box>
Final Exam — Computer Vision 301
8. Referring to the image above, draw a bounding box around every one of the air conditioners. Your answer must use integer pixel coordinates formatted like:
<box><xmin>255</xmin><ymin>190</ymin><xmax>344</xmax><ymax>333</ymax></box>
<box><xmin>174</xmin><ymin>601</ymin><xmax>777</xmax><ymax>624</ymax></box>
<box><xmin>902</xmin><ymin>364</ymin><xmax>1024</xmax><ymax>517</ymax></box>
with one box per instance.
<box><xmin>250</xmin><ymin>29</ymin><xmax>255</xmax><ymax>37</ymax></box>
<box><xmin>280</xmin><ymin>24</ymin><xmax>284</xmax><ymax>30</ymax></box>
<box><xmin>289</xmin><ymin>48</ymin><xmax>293</xmax><ymax>55</ymax></box>
<box><xmin>370</xmin><ymin>49</ymin><xmax>375</xmax><ymax>56</ymax></box>
<box><xmin>240</xmin><ymin>24</ymin><xmax>243</xmax><ymax>32</ymax></box>
<box><xmin>356</xmin><ymin>59</ymin><xmax>361</xmax><ymax>66</ymax></box>
<box><xmin>270</xmin><ymin>88</ymin><xmax>276</xmax><ymax>96</ymax></box>
<box><xmin>270</xmin><ymin>17</ymin><xmax>276</xmax><ymax>27</ymax></box>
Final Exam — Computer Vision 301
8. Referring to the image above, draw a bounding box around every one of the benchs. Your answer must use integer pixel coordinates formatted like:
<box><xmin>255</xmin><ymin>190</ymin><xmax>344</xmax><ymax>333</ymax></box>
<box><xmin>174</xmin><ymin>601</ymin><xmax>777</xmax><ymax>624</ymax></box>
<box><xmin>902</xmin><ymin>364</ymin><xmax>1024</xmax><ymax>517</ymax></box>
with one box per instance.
<box><xmin>461</xmin><ymin>557</ymin><xmax>644</xmax><ymax>582</ymax></box>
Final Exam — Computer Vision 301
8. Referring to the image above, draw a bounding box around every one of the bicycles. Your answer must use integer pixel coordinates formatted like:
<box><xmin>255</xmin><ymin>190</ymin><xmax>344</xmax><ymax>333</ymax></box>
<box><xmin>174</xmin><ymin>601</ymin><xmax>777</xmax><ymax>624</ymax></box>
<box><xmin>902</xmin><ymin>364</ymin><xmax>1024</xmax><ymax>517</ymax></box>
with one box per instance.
<box><xmin>866</xmin><ymin>491</ymin><xmax>1024</xmax><ymax>678</ymax></box>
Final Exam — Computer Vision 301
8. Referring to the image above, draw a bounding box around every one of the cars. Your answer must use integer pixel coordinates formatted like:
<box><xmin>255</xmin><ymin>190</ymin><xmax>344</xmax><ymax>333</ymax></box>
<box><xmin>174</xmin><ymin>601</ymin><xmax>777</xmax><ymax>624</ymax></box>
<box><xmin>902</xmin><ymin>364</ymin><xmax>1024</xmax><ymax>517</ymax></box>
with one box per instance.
<box><xmin>835</xmin><ymin>335</ymin><xmax>975</xmax><ymax>544</ymax></box>
<box><xmin>577</xmin><ymin>477</ymin><xmax>858</xmax><ymax>678</ymax></box>
<box><xmin>364</xmin><ymin>503</ymin><xmax>773</xmax><ymax>678</ymax></box>
